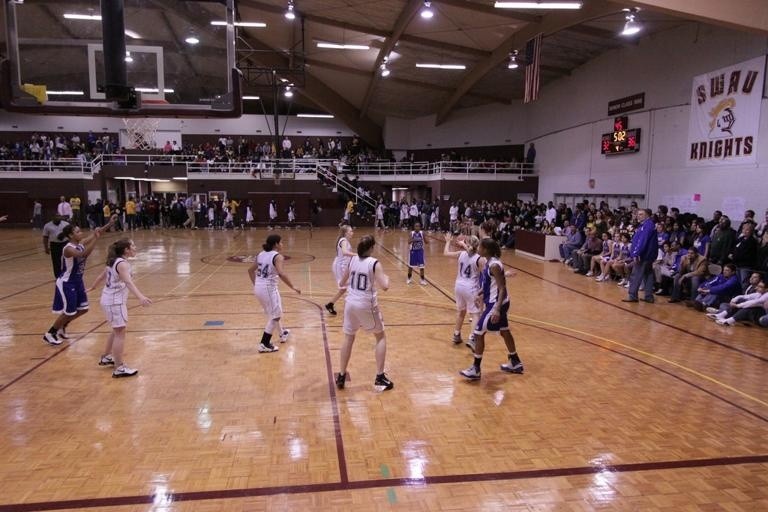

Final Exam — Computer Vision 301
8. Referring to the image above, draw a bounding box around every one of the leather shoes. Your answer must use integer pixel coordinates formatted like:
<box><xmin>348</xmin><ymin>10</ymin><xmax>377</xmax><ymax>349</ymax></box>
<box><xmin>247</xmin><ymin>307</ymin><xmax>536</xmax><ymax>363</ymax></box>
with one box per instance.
<box><xmin>579</xmin><ymin>269</ymin><xmax>588</xmax><ymax>275</ymax></box>
<box><xmin>668</xmin><ymin>298</ymin><xmax>682</xmax><ymax>303</ymax></box>
<box><xmin>574</xmin><ymin>268</ymin><xmax>583</xmax><ymax>273</ymax></box>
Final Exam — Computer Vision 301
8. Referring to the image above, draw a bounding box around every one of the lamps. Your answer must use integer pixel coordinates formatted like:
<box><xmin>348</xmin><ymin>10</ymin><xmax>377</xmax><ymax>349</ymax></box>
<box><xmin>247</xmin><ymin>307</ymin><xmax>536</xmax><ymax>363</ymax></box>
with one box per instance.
<box><xmin>185</xmin><ymin>30</ymin><xmax>200</xmax><ymax>45</ymax></box>
<box><xmin>211</xmin><ymin>20</ymin><xmax>267</xmax><ymax>28</ymax></box>
<box><xmin>508</xmin><ymin>50</ymin><xmax>519</xmax><ymax>69</ymax></box>
<box><xmin>317</xmin><ymin>43</ymin><xmax>370</xmax><ymax>50</ymax></box>
<box><xmin>63</xmin><ymin>13</ymin><xmax>103</xmax><ymax>20</ymax></box>
<box><xmin>380</xmin><ymin>63</ymin><xmax>391</xmax><ymax>77</ymax></box>
<box><xmin>112</xmin><ymin>176</ymin><xmax>187</xmax><ymax>182</ymax></box>
<box><xmin>125</xmin><ymin>49</ymin><xmax>134</xmax><ymax>63</ymax></box>
<box><xmin>619</xmin><ymin>7</ymin><xmax>643</xmax><ymax>37</ymax></box>
<box><xmin>420</xmin><ymin>0</ymin><xmax>434</xmax><ymax>20</ymax></box>
<box><xmin>45</xmin><ymin>89</ymin><xmax>84</xmax><ymax>96</ymax></box>
<box><xmin>416</xmin><ymin>62</ymin><xmax>467</xmax><ymax>70</ymax></box>
<box><xmin>284</xmin><ymin>87</ymin><xmax>294</xmax><ymax>97</ymax></box>
<box><xmin>297</xmin><ymin>114</ymin><xmax>335</xmax><ymax>119</ymax></box>
<box><xmin>217</xmin><ymin>94</ymin><xmax>260</xmax><ymax>100</ymax></box>
<box><xmin>285</xmin><ymin>0</ymin><xmax>296</xmax><ymax>20</ymax></box>
<box><xmin>135</xmin><ymin>87</ymin><xmax>174</xmax><ymax>93</ymax></box>
<box><xmin>494</xmin><ymin>2</ymin><xmax>582</xmax><ymax>9</ymax></box>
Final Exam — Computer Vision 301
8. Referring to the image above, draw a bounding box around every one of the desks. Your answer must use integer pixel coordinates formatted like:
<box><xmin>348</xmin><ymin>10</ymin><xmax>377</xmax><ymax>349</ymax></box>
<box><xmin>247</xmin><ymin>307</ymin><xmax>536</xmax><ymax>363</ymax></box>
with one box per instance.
<box><xmin>515</xmin><ymin>229</ymin><xmax>568</xmax><ymax>262</ymax></box>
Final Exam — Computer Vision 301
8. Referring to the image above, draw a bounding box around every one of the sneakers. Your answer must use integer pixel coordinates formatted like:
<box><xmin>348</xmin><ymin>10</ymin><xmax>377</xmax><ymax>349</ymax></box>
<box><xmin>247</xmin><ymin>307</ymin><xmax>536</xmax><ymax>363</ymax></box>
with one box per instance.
<box><xmin>623</xmin><ymin>281</ymin><xmax>630</xmax><ymax>288</ymax></box>
<box><xmin>617</xmin><ymin>279</ymin><xmax>627</xmax><ymax>286</ymax></box>
<box><xmin>685</xmin><ymin>299</ymin><xmax>695</xmax><ymax>307</ymax></box>
<box><xmin>716</xmin><ymin>318</ymin><xmax>735</xmax><ymax>327</ymax></box>
<box><xmin>336</xmin><ymin>373</ymin><xmax>347</xmax><ymax>389</ymax></box>
<box><xmin>639</xmin><ymin>280</ymin><xmax>645</xmax><ymax>290</ymax></box>
<box><xmin>706</xmin><ymin>313</ymin><xmax>722</xmax><ymax>320</ymax></box>
<box><xmin>279</xmin><ymin>329</ymin><xmax>290</xmax><ymax>343</ymax></box>
<box><xmin>325</xmin><ymin>303</ymin><xmax>337</xmax><ymax>315</ymax></box>
<box><xmin>406</xmin><ymin>279</ymin><xmax>412</xmax><ymax>285</ymax></box>
<box><xmin>375</xmin><ymin>373</ymin><xmax>393</xmax><ymax>391</ymax></box>
<box><xmin>459</xmin><ymin>366</ymin><xmax>482</xmax><ymax>379</ymax></box>
<box><xmin>98</xmin><ymin>353</ymin><xmax>114</xmax><ymax>365</ymax></box>
<box><xmin>621</xmin><ymin>297</ymin><xmax>639</xmax><ymax>302</ymax></box>
<box><xmin>604</xmin><ymin>275</ymin><xmax>610</xmax><ymax>280</ymax></box>
<box><xmin>112</xmin><ymin>365</ymin><xmax>139</xmax><ymax>378</ymax></box>
<box><xmin>464</xmin><ymin>336</ymin><xmax>474</xmax><ymax>352</ymax></box>
<box><xmin>500</xmin><ymin>358</ymin><xmax>524</xmax><ymax>373</ymax></box>
<box><xmin>654</xmin><ymin>289</ymin><xmax>669</xmax><ymax>295</ymax></box>
<box><xmin>595</xmin><ymin>278</ymin><xmax>604</xmax><ymax>282</ymax></box>
<box><xmin>640</xmin><ymin>297</ymin><xmax>654</xmax><ymax>304</ymax></box>
<box><xmin>419</xmin><ymin>280</ymin><xmax>427</xmax><ymax>286</ymax></box>
<box><xmin>452</xmin><ymin>332</ymin><xmax>463</xmax><ymax>344</ymax></box>
<box><xmin>707</xmin><ymin>306</ymin><xmax>720</xmax><ymax>314</ymax></box>
<box><xmin>586</xmin><ymin>272</ymin><xmax>594</xmax><ymax>277</ymax></box>
<box><xmin>42</xmin><ymin>332</ymin><xmax>64</xmax><ymax>345</ymax></box>
<box><xmin>257</xmin><ymin>343</ymin><xmax>280</xmax><ymax>353</ymax></box>
<box><xmin>58</xmin><ymin>328</ymin><xmax>71</xmax><ymax>339</ymax></box>
<box><xmin>694</xmin><ymin>300</ymin><xmax>709</xmax><ymax>312</ymax></box>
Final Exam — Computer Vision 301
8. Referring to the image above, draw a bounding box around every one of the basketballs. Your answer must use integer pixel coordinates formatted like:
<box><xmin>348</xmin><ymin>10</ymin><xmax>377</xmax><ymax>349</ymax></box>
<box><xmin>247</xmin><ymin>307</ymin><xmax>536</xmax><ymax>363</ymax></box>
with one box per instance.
<box><xmin>455</xmin><ymin>235</ymin><xmax>466</xmax><ymax>248</ymax></box>
